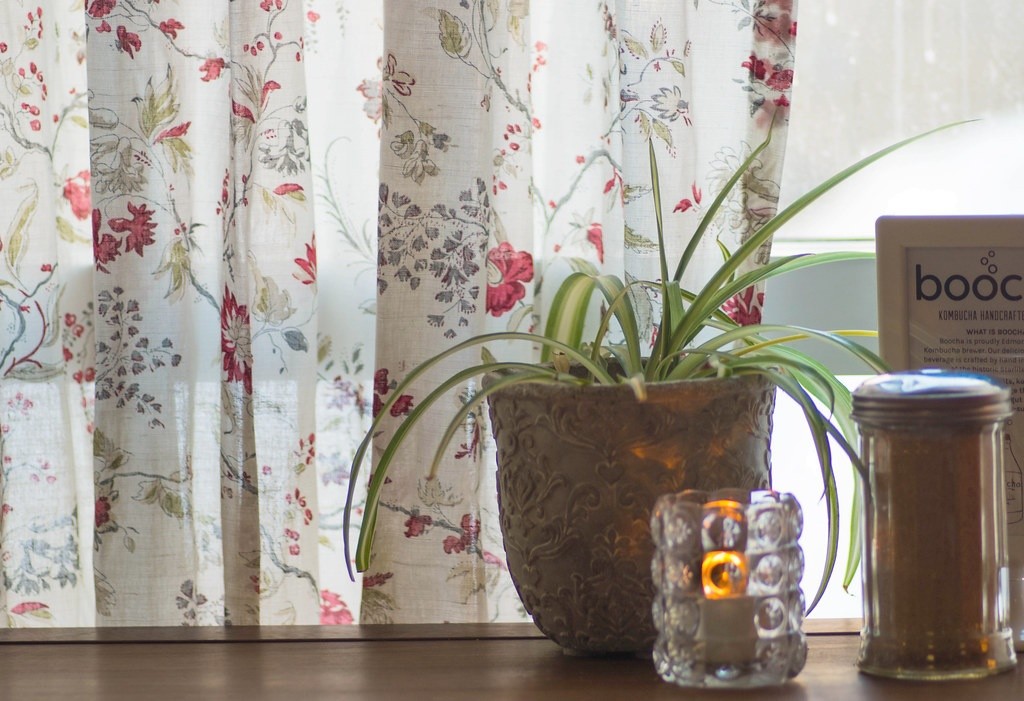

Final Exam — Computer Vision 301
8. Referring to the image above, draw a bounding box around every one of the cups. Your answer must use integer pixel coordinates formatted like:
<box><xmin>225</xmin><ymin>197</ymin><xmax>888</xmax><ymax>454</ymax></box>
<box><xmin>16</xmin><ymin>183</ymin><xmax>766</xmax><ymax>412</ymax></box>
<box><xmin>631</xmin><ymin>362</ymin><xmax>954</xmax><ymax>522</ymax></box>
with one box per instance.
<box><xmin>649</xmin><ymin>488</ymin><xmax>811</xmax><ymax>691</ymax></box>
<box><xmin>849</xmin><ymin>370</ymin><xmax>1019</xmax><ymax>682</ymax></box>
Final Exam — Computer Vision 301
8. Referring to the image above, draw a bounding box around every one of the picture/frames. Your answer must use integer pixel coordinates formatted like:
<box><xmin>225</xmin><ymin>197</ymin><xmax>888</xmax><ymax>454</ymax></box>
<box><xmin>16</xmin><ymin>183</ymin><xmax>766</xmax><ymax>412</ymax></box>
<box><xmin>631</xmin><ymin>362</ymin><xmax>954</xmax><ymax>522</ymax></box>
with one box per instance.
<box><xmin>876</xmin><ymin>216</ymin><xmax>1024</xmax><ymax>651</ymax></box>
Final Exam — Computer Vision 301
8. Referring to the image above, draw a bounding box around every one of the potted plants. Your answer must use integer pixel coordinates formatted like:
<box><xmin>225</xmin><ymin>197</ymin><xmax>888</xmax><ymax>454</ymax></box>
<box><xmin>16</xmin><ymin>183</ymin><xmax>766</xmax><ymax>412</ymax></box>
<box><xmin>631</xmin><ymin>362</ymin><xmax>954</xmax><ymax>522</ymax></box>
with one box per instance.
<box><xmin>341</xmin><ymin>99</ymin><xmax>983</xmax><ymax>663</ymax></box>
